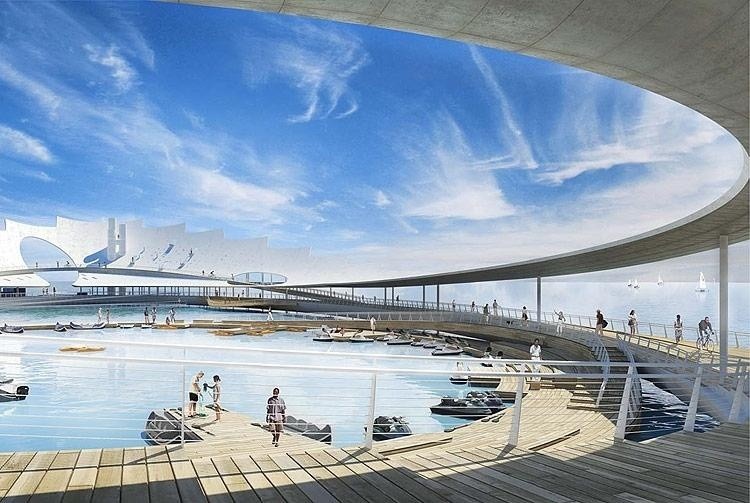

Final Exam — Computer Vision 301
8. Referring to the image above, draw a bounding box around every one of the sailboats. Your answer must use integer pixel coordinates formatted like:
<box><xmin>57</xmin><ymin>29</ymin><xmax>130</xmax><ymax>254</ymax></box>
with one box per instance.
<box><xmin>695</xmin><ymin>272</ymin><xmax>709</xmax><ymax>292</ymax></box>
<box><xmin>657</xmin><ymin>272</ymin><xmax>664</xmax><ymax>286</ymax></box>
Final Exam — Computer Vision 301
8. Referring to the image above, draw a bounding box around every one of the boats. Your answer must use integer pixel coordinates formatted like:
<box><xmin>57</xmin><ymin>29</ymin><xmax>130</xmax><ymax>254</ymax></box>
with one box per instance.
<box><xmin>252</xmin><ymin>416</ymin><xmax>331</xmax><ymax>445</ymax></box>
<box><xmin>1</xmin><ymin>326</ymin><xmax>24</xmax><ymax>333</ymax></box>
<box><xmin>312</xmin><ymin>325</ymin><xmax>465</xmax><ymax>355</ymax></box>
<box><xmin>0</xmin><ymin>379</ymin><xmax>29</xmax><ymax>402</ymax></box>
<box><xmin>450</xmin><ymin>366</ymin><xmax>468</xmax><ymax>384</ymax></box>
<box><xmin>628</xmin><ymin>279</ymin><xmax>639</xmax><ymax>288</ymax></box>
<box><xmin>364</xmin><ymin>415</ymin><xmax>413</xmax><ymax>440</ymax></box>
<box><xmin>144</xmin><ymin>411</ymin><xmax>187</xmax><ymax>445</ymax></box>
<box><xmin>430</xmin><ymin>391</ymin><xmax>506</xmax><ymax>415</ymax></box>
<box><xmin>54</xmin><ymin>321</ymin><xmax>105</xmax><ymax>331</ymax></box>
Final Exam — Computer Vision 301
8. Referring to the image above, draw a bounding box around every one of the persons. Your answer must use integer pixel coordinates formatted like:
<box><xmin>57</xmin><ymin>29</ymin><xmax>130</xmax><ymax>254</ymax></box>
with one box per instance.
<box><xmin>188</xmin><ymin>371</ymin><xmax>204</xmax><ymax>417</ymax></box>
<box><xmin>698</xmin><ymin>317</ymin><xmax>714</xmax><ymax>345</ymax></box>
<box><xmin>168</xmin><ymin>308</ymin><xmax>176</xmax><ymax>324</ymax></box>
<box><xmin>673</xmin><ymin>314</ymin><xmax>682</xmax><ymax>343</ymax></box>
<box><xmin>493</xmin><ymin>351</ymin><xmax>506</xmax><ymax>367</ymax></box>
<box><xmin>595</xmin><ymin>309</ymin><xmax>603</xmax><ymax>336</ymax></box>
<box><xmin>530</xmin><ymin>338</ymin><xmax>542</xmax><ymax>370</ymax></box>
<box><xmin>202</xmin><ymin>270</ymin><xmax>262</xmax><ymax>301</ymax></box>
<box><xmin>266</xmin><ymin>388</ymin><xmax>287</xmax><ymax>447</ymax></box>
<box><xmin>320</xmin><ymin>324</ymin><xmax>344</xmax><ymax>338</ymax></box>
<box><xmin>105</xmin><ymin>308</ymin><xmax>109</xmax><ymax>324</ymax></box>
<box><xmin>166</xmin><ymin>316</ymin><xmax>171</xmax><ymax>326</ymax></box>
<box><xmin>470</xmin><ymin>300</ymin><xmax>501</xmax><ymax>322</ymax></box>
<box><xmin>150</xmin><ymin>306</ymin><xmax>156</xmax><ymax>324</ymax></box>
<box><xmin>203</xmin><ymin>375</ymin><xmax>222</xmax><ymax>422</ymax></box>
<box><xmin>266</xmin><ymin>305</ymin><xmax>273</xmax><ymax>321</ymax></box>
<box><xmin>484</xmin><ymin>347</ymin><xmax>493</xmax><ymax>364</ymax></box>
<box><xmin>628</xmin><ymin>310</ymin><xmax>637</xmax><ymax>336</ymax></box>
<box><xmin>144</xmin><ymin>307</ymin><xmax>150</xmax><ymax>325</ymax></box>
<box><xmin>519</xmin><ymin>306</ymin><xmax>528</xmax><ymax>328</ymax></box>
<box><xmin>452</xmin><ymin>300</ymin><xmax>456</xmax><ymax>311</ymax></box>
<box><xmin>554</xmin><ymin>310</ymin><xmax>566</xmax><ymax>337</ymax></box>
<box><xmin>99</xmin><ymin>307</ymin><xmax>103</xmax><ymax>322</ymax></box>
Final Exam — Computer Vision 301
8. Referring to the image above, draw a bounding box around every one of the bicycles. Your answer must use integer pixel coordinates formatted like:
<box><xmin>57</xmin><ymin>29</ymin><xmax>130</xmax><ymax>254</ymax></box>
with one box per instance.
<box><xmin>696</xmin><ymin>333</ymin><xmax>715</xmax><ymax>352</ymax></box>
